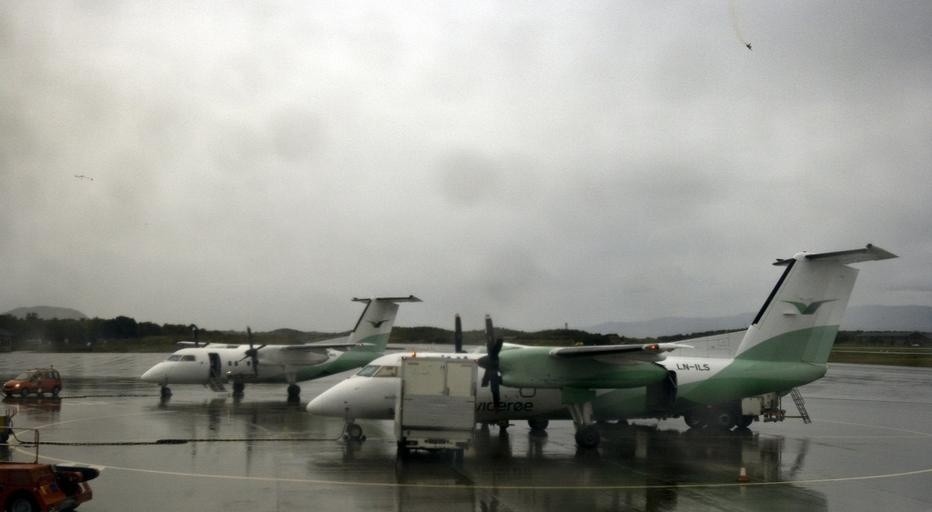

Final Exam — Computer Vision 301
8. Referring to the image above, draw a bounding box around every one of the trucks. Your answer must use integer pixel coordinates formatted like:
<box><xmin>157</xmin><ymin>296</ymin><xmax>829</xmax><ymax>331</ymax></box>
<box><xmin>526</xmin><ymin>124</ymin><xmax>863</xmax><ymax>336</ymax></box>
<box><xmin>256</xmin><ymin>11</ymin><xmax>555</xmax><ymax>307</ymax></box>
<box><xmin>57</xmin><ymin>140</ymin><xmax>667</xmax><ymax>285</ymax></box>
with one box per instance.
<box><xmin>393</xmin><ymin>351</ymin><xmax>479</xmax><ymax>464</ymax></box>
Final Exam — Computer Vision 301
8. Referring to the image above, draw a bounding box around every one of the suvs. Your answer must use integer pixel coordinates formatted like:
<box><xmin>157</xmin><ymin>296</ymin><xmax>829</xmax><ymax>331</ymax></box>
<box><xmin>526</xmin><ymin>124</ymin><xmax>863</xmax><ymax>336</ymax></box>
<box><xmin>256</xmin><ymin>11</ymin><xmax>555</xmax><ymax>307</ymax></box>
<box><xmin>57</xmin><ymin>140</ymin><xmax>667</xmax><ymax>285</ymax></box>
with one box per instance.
<box><xmin>3</xmin><ymin>368</ymin><xmax>63</xmax><ymax>397</ymax></box>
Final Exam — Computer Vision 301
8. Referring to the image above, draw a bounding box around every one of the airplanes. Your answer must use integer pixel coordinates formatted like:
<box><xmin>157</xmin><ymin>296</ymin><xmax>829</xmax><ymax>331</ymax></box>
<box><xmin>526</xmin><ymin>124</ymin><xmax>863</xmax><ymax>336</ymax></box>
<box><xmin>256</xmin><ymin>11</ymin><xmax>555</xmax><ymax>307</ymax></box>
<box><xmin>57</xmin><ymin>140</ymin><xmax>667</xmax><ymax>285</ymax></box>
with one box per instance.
<box><xmin>304</xmin><ymin>244</ymin><xmax>899</xmax><ymax>444</ymax></box>
<box><xmin>140</xmin><ymin>294</ymin><xmax>425</xmax><ymax>404</ymax></box>
